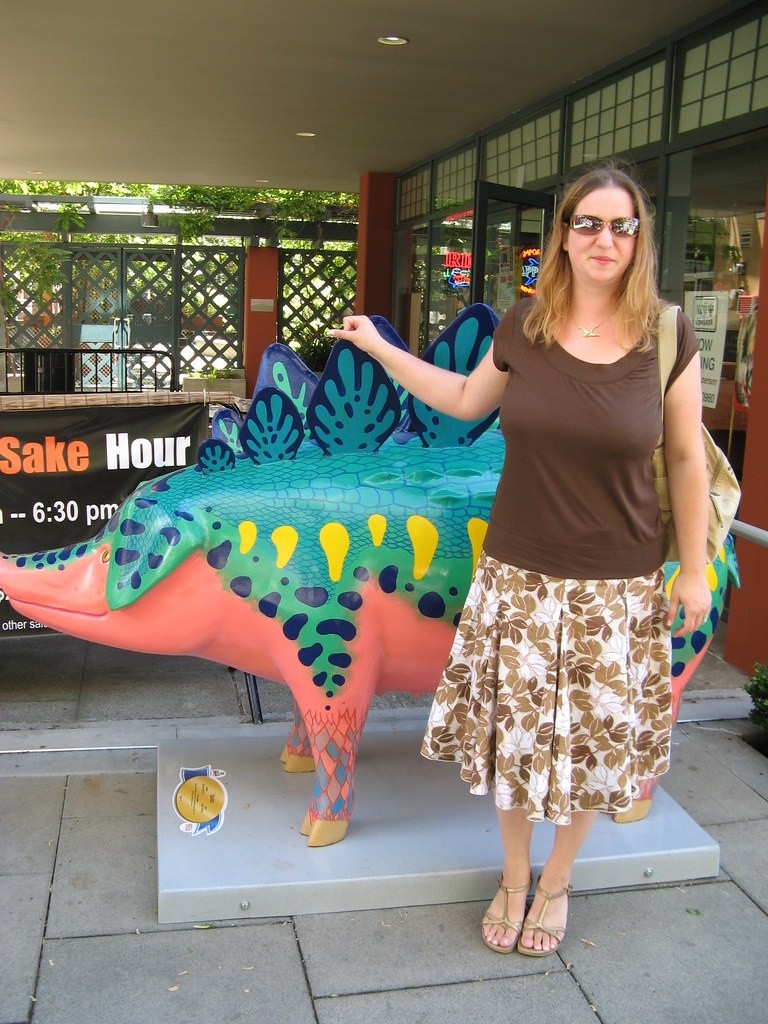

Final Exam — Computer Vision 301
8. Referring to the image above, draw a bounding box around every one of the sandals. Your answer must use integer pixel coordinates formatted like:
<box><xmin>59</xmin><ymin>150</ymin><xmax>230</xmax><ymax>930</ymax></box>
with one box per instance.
<box><xmin>481</xmin><ymin>871</ymin><xmax>534</xmax><ymax>954</ymax></box>
<box><xmin>517</xmin><ymin>874</ymin><xmax>573</xmax><ymax>957</ymax></box>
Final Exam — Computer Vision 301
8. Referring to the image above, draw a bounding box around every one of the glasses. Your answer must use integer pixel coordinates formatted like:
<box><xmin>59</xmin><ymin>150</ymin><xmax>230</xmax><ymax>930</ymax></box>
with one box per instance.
<box><xmin>566</xmin><ymin>214</ymin><xmax>640</xmax><ymax>239</ymax></box>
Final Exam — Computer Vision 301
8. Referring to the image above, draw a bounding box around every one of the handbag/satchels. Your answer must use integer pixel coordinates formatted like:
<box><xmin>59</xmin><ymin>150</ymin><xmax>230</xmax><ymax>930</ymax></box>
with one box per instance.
<box><xmin>653</xmin><ymin>307</ymin><xmax>742</xmax><ymax>564</ymax></box>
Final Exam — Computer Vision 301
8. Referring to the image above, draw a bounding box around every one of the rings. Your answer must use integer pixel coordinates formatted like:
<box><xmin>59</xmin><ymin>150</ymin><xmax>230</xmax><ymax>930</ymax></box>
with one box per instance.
<box><xmin>703</xmin><ymin>617</ymin><xmax>708</xmax><ymax>623</ymax></box>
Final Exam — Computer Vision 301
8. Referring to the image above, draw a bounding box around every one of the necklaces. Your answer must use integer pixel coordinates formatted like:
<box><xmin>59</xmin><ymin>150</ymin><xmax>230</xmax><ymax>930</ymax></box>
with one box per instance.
<box><xmin>570</xmin><ymin>310</ymin><xmax>610</xmax><ymax>337</ymax></box>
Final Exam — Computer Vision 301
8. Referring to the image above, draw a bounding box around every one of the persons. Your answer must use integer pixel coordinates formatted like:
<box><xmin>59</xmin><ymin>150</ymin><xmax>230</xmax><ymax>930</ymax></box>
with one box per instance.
<box><xmin>328</xmin><ymin>169</ymin><xmax>711</xmax><ymax>957</ymax></box>
